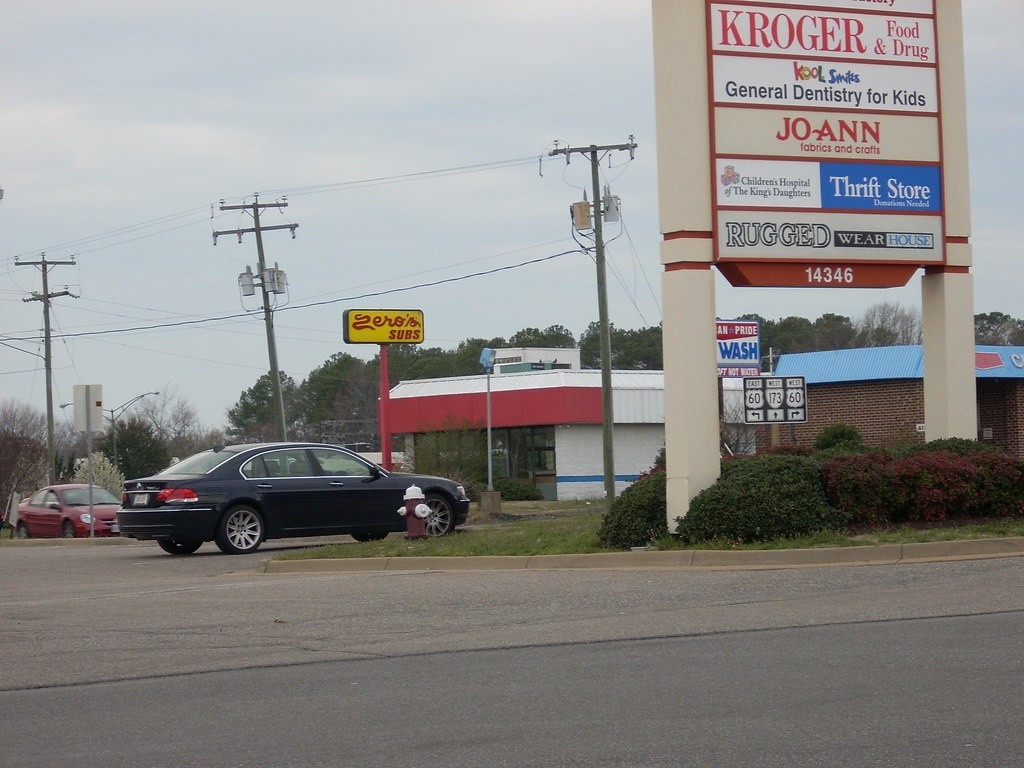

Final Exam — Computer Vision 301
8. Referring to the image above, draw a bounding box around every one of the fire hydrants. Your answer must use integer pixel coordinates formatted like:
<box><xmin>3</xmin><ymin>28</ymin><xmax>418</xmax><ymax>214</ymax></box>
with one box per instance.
<box><xmin>397</xmin><ymin>485</ymin><xmax>433</xmax><ymax>540</ymax></box>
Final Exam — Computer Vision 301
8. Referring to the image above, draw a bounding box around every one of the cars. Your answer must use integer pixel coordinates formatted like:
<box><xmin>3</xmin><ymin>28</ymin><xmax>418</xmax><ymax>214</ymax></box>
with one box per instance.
<box><xmin>115</xmin><ymin>440</ymin><xmax>471</xmax><ymax>555</ymax></box>
<box><xmin>16</xmin><ymin>483</ymin><xmax>123</xmax><ymax>539</ymax></box>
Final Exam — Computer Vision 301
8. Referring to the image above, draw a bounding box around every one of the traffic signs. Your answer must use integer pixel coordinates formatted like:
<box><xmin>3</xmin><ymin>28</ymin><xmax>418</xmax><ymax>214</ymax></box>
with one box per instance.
<box><xmin>742</xmin><ymin>376</ymin><xmax>807</xmax><ymax>424</ymax></box>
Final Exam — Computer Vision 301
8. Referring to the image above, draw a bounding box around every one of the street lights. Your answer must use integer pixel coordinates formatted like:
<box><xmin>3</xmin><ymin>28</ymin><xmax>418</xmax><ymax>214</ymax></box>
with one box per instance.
<box><xmin>59</xmin><ymin>391</ymin><xmax>160</xmax><ymax>471</ymax></box>
<box><xmin>480</xmin><ymin>347</ymin><xmax>498</xmax><ymax>491</ymax></box>
